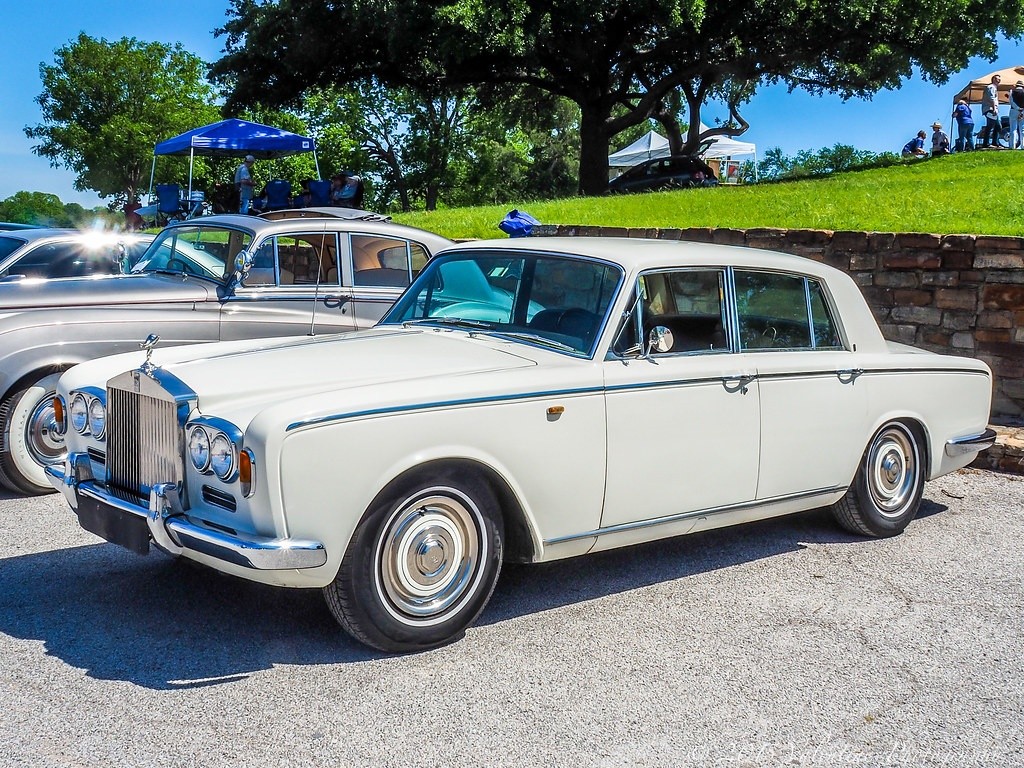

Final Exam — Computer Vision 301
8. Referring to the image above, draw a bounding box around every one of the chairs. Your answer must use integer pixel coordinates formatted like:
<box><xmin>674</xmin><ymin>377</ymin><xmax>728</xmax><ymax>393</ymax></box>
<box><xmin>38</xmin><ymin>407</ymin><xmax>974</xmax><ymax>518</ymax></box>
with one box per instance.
<box><xmin>289</xmin><ymin>180</ymin><xmax>332</xmax><ymax>207</ymax></box>
<box><xmin>251</xmin><ymin>179</ymin><xmax>290</xmax><ymax>214</ymax></box>
<box><xmin>535</xmin><ymin>308</ymin><xmax>601</xmax><ymax>337</ymax></box>
<box><xmin>154</xmin><ymin>184</ymin><xmax>204</xmax><ymax>227</ymax></box>
<box><xmin>122</xmin><ymin>203</ymin><xmax>149</xmax><ymax>230</ymax></box>
<box><xmin>335</xmin><ymin>179</ymin><xmax>365</xmax><ymax>209</ymax></box>
<box><xmin>642</xmin><ymin>315</ymin><xmax>723</xmax><ymax>352</ymax></box>
<box><xmin>245</xmin><ymin>268</ymin><xmax>294</xmax><ymax>284</ymax></box>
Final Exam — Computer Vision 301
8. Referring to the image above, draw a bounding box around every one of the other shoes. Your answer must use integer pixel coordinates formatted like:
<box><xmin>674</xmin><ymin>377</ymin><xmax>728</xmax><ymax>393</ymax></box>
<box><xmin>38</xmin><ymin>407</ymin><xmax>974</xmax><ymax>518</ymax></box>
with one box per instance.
<box><xmin>998</xmin><ymin>146</ymin><xmax>1004</xmax><ymax>149</ymax></box>
<box><xmin>988</xmin><ymin>144</ymin><xmax>997</xmax><ymax>148</ymax></box>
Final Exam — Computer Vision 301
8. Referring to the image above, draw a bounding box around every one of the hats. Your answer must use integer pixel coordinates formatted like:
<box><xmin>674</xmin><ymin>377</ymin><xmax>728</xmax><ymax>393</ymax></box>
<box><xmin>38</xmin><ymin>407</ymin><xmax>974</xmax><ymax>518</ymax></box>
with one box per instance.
<box><xmin>348</xmin><ymin>176</ymin><xmax>361</xmax><ymax>182</ymax></box>
<box><xmin>930</xmin><ymin>122</ymin><xmax>942</xmax><ymax>129</ymax></box>
<box><xmin>958</xmin><ymin>100</ymin><xmax>966</xmax><ymax>105</ymax></box>
<box><xmin>1013</xmin><ymin>80</ymin><xmax>1024</xmax><ymax>88</ymax></box>
<box><xmin>246</xmin><ymin>155</ymin><xmax>255</xmax><ymax>162</ymax></box>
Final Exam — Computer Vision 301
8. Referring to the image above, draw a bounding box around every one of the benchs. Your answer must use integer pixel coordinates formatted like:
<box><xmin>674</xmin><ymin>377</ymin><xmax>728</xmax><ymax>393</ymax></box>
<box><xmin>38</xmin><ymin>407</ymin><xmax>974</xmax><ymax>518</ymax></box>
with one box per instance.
<box><xmin>355</xmin><ymin>268</ymin><xmax>408</xmax><ymax>286</ymax></box>
<box><xmin>740</xmin><ymin>315</ymin><xmax>828</xmax><ymax>346</ymax></box>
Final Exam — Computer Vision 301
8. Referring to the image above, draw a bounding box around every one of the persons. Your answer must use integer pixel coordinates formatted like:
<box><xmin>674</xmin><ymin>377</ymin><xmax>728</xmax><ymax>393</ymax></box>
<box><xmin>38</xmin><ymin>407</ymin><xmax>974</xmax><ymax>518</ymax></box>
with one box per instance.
<box><xmin>1009</xmin><ymin>80</ymin><xmax>1024</xmax><ymax>150</ymax></box>
<box><xmin>929</xmin><ymin>122</ymin><xmax>950</xmax><ymax>154</ymax></box>
<box><xmin>616</xmin><ymin>167</ymin><xmax>624</xmax><ymax>177</ymax></box>
<box><xmin>981</xmin><ymin>75</ymin><xmax>1004</xmax><ymax>150</ymax></box>
<box><xmin>332</xmin><ymin>170</ymin><xmax>362</xmax><ymax>201</ymax></box>
<box><xmin>235</xmin><ymin>154</ymin><xmax>258</xmax><ymax>214</ymax></box>
<box><xmin>902</xmin><ymin>130</ymin><xmax>926</xmax><ymax>159</ymax></box>
<box><xmin>952</xmin><ymin>100</ymin><xmax>975</xmax><ymax>153</ymax></box>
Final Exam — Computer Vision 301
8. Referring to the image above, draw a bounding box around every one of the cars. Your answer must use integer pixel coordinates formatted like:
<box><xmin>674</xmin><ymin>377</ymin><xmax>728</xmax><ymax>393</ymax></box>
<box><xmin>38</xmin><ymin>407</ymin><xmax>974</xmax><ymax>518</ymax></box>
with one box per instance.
<box><xmin>42</xmin><ymin>235</ymin><xmax>1003</xmax><ymax>657</ymax></box>
<box><xmin>608</xmin><ymin>153</ymin><xmax>720</xmax><ymax>195</ymax></box>
<box><xmin>0</xmin><ymin>219</ymin><xmax>234</xmax><ymax>288</ymax></box>
<box><xmin>1</xmin><ymin>219</ymin><xmax>553</xmax><ymax>495</ymax></box>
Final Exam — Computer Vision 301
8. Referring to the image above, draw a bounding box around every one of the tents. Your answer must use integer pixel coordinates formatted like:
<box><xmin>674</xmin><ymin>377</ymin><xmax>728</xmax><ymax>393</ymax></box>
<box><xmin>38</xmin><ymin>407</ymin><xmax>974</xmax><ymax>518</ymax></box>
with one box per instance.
<box><xmin>681</xmin><ymin>122</ymin><xmax>757</xmax><ymax>183</ymax></box>
<box><xmin>950</xmin><ymin>66</ymin><xmax>1024</xmax><ymax>154</ymax></box>
<box><xmin>146</xmin><ymin>117</ymin><xmax>322</xmax><ymax>220</ymax></box>
<box><xmin>608</xmin><ymin>130</ymin><xmax>671</xmax><ymax>167</ymax></box>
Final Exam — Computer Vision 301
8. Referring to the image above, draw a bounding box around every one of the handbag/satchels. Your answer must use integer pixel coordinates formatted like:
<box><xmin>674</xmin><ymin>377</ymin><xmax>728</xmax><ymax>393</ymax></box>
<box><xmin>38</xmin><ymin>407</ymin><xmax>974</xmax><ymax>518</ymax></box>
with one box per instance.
<box><xmin>499</xmin><ymin>209</ymin><xmax>541</xmax><ymax>238</ymax></box>
